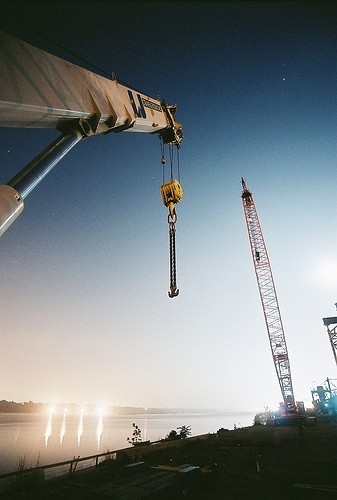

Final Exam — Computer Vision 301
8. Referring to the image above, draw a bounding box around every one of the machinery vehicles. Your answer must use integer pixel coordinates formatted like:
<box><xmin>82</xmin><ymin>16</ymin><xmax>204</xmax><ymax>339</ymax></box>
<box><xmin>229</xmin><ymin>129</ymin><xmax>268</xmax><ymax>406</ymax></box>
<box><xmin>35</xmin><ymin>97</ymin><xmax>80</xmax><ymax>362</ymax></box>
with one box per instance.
<box><xmin>1</xmin><ymin>32</ymin><xmax>185</xmax><ymax>303</ymax></box>
